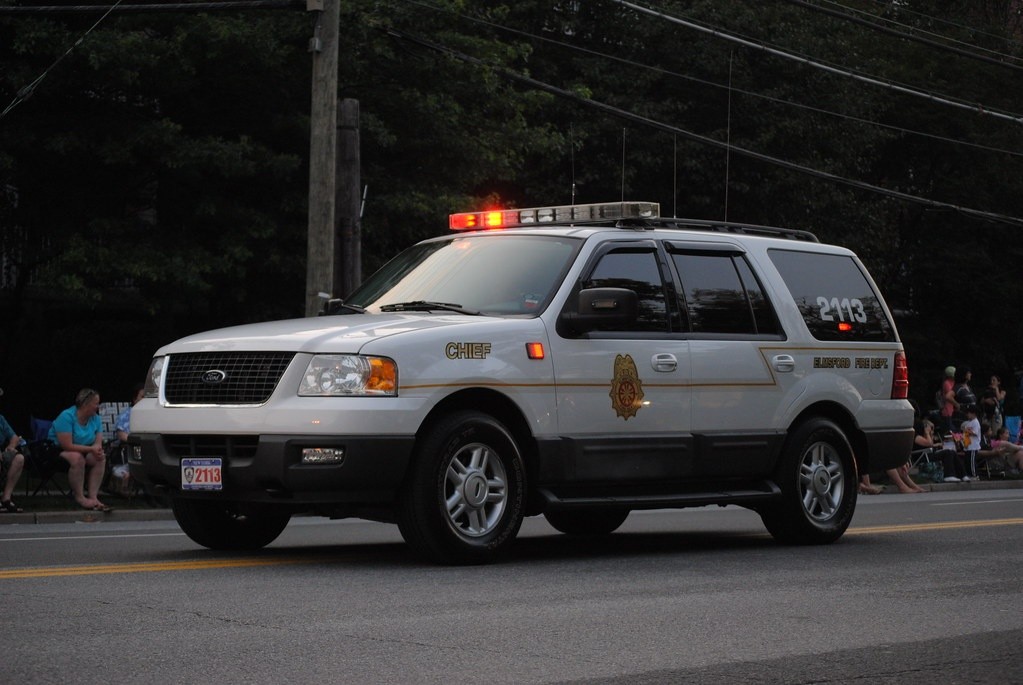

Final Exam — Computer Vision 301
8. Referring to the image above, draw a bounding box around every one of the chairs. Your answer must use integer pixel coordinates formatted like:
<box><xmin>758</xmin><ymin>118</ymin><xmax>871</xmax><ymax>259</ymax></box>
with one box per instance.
<box><xmin>909</xmin><ymin>443</ymin><xmax>994</xmax><ymax>478</ymax></box>
<box><xmin>15</xmin><ymin>417</ymin><xmax>142</xmax><ymax>504</ymax></box>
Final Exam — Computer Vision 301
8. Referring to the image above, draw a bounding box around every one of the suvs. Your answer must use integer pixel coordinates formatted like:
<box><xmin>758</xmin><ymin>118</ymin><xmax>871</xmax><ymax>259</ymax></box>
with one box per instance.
<box><xmin>128</xmin><ymin>200</ymin><xmax>914</xmax><ymax>566</ymax></box>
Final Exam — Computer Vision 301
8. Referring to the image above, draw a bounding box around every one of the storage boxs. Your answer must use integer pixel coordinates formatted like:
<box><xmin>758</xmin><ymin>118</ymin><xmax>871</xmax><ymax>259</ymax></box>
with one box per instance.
<box><xmin>99</xmin><ymin>402</ymin><xmax>131</xmax><ymax>441</ymax></box>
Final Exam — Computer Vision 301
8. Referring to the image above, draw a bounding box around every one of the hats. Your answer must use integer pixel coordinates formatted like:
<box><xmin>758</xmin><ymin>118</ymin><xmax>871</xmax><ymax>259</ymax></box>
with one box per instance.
<box><xmin>945</xmin><ymin>366</ymin><xmax>956</xmax><ymax>377</ymax></box>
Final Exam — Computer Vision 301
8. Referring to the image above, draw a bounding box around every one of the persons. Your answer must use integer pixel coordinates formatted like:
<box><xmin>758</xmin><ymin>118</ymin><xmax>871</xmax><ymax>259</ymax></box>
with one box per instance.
<box><xmin>0</xmin><ymin>414</ymin><xmax>25</xmax><ymax>513</ymax></box>
<box><xmin>859</xmin><ymin>366</ymin><xmax>1023</xmax><ymax>495</ymax></box>
<box><xmin>42</xmin><ymin>388</ymin><xmax>114</xmax><ymax>512</ymax></box>
<box><xmin>116</xmin><ymin>384</ymin><xmax>145</xmax><ymax>441</ymax></box>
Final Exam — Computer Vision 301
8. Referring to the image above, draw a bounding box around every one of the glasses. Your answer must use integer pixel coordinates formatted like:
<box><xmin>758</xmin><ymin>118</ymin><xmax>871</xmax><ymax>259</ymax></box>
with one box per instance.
<box><xmin>81</xmin><ymin>390</ymin><xmax>94</xmax><ymax>403</ymax></box>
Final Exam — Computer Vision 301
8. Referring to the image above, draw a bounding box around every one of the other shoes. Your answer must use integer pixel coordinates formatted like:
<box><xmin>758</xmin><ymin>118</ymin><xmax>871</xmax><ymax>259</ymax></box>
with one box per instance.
<box><xmin>970</xmin><ymin>476</ymin><xmax>980</xmax><ymax>481</ymax></box>
<box><xmin>861</xmin><ymin>483</ymin><xmax>880</xmax><ymax>495</ymax></box>
<box><xmin>962</xmin><ymin>476</ymin><xmax>971</xmax><ymax>482</ymax></box>
<box><xmin>944</xmin><ymin>476</ymin><xmax>961</xmax><ymax>482</ymax></box>
<box><xmin>77</xmin><ymin>498</ymin><xmax>114</xmax><ymax>511</ymax></box>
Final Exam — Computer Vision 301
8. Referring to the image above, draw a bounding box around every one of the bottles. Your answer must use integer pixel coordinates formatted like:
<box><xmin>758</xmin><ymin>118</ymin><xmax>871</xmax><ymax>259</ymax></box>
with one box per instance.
<box><xmin>19</xmin><ymin>436</ymin><xmax>30</xmax><ymax>456</ymax></box>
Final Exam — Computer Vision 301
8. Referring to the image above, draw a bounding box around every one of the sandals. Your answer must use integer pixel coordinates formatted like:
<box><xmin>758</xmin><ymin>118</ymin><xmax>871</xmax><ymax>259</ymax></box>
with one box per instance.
<box><xmin>0</xmin><ymin>497</ymin><xmax>23</xmax><ymax>513</ymax></box>
